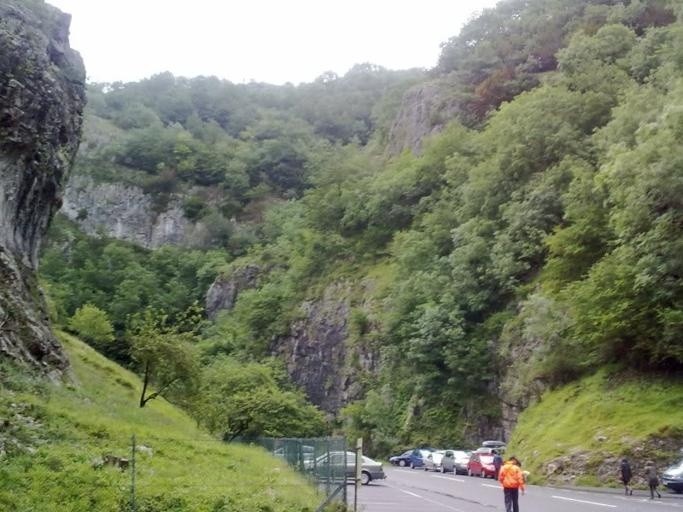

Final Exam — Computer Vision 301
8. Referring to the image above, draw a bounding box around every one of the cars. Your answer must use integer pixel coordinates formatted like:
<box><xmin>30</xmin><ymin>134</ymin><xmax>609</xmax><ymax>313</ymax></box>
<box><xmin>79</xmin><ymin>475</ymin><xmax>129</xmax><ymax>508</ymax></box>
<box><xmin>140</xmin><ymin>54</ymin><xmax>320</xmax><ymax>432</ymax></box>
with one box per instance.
<box><xmin>389</xmin><ymin>440</ymin><xmax>507</xmax><ymax>481</ymax></box>
<box><xmin>660</xmin><ymin>461</ymin><xmax>682</xmax><ymax>492</ymax></box>
<box><xmin>268</xmin><ymin>445</ymin><xmax>387</xmax><ymax>486</ymax></box>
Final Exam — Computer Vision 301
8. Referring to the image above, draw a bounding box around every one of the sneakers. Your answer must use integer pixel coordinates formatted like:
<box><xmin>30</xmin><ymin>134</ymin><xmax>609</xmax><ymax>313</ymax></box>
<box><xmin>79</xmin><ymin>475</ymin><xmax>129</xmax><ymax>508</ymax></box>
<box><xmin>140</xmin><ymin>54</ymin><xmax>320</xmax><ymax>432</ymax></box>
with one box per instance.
<box><xmin>624</xmin><ymin>488</ymin><xmax>663</xmax><ymax>499</ymax></box>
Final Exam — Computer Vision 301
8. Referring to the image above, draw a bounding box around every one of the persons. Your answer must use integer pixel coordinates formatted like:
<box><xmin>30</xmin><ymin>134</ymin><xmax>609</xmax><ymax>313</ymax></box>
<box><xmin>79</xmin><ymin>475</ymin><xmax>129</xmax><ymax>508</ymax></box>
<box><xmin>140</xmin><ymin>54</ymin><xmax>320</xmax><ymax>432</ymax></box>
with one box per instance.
<box><xmin>492</xmin><ymin>451</ymin><xmax>503</xmax><ymax>481</ymax></box>
<box><xmin>618</xmin><ymin>457</ymin><xmax>632</xmax><ymax>496</ymax></box>
<box><xmin>497</xmin><ymin>453</ymin><xmax>526</xmax><ymax>511</ymax></box>
<box><xmin>641</xmin><ymin>459</ymin><xmax>661</xmax><ymax>499</ymax></box>
